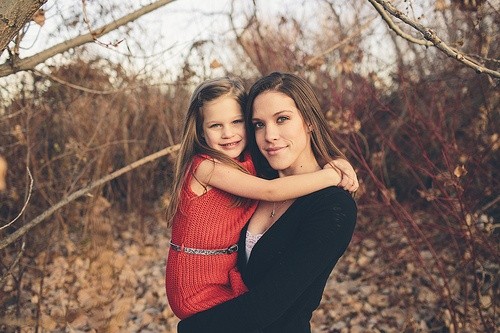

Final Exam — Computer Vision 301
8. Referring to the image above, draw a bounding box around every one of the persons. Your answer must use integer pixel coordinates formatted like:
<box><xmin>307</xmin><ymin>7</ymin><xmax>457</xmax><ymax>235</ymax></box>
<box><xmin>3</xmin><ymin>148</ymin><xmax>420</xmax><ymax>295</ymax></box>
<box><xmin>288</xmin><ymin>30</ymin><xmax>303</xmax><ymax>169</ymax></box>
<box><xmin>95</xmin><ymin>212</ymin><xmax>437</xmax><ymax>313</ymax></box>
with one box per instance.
<box><xmin>164</xmin><ymin>75</ymin><xmax>358</xmax><ymax>321</ymax></box>
<box><xmin>172</xmin><ymin>70</ymin><xmax>358</xmax><ymax>332</ymax></box>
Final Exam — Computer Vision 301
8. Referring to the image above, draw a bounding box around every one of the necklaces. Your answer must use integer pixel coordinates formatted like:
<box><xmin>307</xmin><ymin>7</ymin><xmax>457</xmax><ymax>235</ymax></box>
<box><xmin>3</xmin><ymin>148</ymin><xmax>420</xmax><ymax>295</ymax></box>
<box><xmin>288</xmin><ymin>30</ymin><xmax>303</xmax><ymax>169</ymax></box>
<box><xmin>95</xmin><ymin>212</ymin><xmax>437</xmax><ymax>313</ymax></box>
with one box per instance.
<box><xmin>265</xmin><ymin>169</ymin><xmax>321</xmax><ymax>217</ymax></box>
